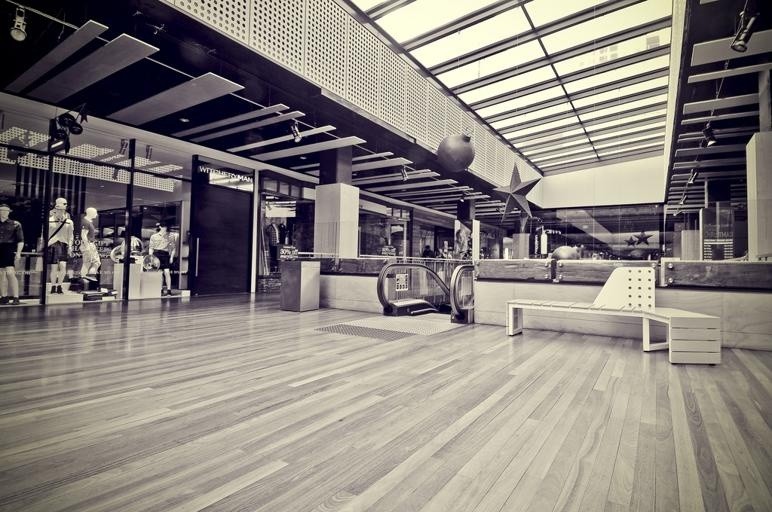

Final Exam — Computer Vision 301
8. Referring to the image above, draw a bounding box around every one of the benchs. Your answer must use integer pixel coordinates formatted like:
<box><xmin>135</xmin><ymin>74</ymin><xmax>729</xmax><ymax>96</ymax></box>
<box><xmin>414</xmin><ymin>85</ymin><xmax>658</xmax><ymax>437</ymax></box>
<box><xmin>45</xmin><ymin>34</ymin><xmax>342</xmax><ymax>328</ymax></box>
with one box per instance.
<box><xmin>506</xmin><ymin>266</ymin><xmax>721</xmax><ymax>366</ymax></box>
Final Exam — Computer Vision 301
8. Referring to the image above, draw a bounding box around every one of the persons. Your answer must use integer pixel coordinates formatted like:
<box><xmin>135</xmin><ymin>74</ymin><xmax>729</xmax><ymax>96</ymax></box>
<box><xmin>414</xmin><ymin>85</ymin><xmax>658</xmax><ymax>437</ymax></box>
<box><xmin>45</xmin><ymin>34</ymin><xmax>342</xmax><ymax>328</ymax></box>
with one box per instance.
<box><xmin>78</xmin><ymin>206</ymin><xmax>102</xmax><ymax>282</ymax></box>
<box><xmin>420</xmin><ymin>245</ymin><xmax>438</xmax><ymax>280</ymax></box>
<box><xmin>48</xmin><ymin>195</ymin><xmax>72</xmax><ymax>283</ymax></box>
<box><xmin>0</xmin><ymin>203</ymin><xmax>25</xmax><ymax>307</ymax></box>
<box><xmin>276</xmin><ymin>219</ymin><xmax>287</xmax><ymax>245</ymax></box>
<box><xmin>266</xmin><ymin>220</ymin><xmax>281</xmax><ymax>272</ymax></box>
<box><xmin>455</xmin><ymin>230</ymin><xmax>469</xmax><ymax>254</ymax></box>
<box><xmin>147</xmin><ymin>221</ymin><xmax>178</xmax><ymax>296</ymax></box>
<box><xmin>39</xmin><ymin>201</ymin><xmax>74</xmax><ymax>294</ymax></box>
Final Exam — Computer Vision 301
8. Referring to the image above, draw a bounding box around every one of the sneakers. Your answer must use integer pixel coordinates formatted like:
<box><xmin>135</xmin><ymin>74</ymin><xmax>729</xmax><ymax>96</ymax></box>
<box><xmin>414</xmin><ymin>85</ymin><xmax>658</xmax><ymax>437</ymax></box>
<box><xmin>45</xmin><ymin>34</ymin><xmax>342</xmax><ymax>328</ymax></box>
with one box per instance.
<box><xmin>50</xmin><ymin>284</ymin><xmax>64</xmax><ymax>295</ymax></box>
<box><xmin>161</xmin><ymin>288</ymin><xmax>172</xmax><ymax>297</ymax></box>
<box><xmin>0</xmin><ymin>296</ymin><xmax>20</xmax><ymax>305</ymax></box>
<box><xmin>81</xmin><ymin>273</ymin><xmax>99</xmax><ymax>283</ymax></box>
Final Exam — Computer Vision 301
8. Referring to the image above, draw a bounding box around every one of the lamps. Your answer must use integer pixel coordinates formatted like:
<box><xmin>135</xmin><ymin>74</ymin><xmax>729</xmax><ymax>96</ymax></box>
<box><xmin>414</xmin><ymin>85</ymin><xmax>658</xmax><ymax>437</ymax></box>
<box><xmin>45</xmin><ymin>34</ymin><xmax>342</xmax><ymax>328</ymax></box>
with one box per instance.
<box><xmin>119</xmin><ymin>139</ymin><xmax>128</xmax><ymax>155</ymax></box>
<box><xmin>702</xmin><ymin>122</ymin><xmax>717</xmax><ymax>146</ymax></box>
<box><xmin>288</xmin><ymin>121</ymin><xmax>302</xmax><ymax>143</ymax></box>
<box><xmin>145</xmin><ymin>145</ymin><xmax>152</xmax><ymax>159</ymax></box>
<box><xmin>730</xmin><ymin>11</ymin><xmax>760</xmax><ymax>52</ymax></box>
<box><xmin>11</xmin><ymin>5</ymin><xmax>27</xmax><ymax>41</ymax></box>
<box><xmin>69</xmin><ymin>123</ymin><xmax>83</xmax><ymax>134</ymax></box>
<box><xmin>400</xmin><ymin>165</ymin><xmax>408</xmax><ymax>181</ymax></box>
<box><xmin>460</xmin><ymin>194</ymin><xmax>466</xmax><ymax>203</ymax></box>
<box><xmin>58</xmin><ymin>113</ymin><xmax>76</xmax><ymax>127</ymax></box>
<box><xmin>688</xmin><ymin>169</ymin><xmax>698</xmax><ymax>184</ymax></box>
<box><xmin>673</xmin><ymin>195</ymin><xmax>688</xmax><ymax>217</ymax></box>
